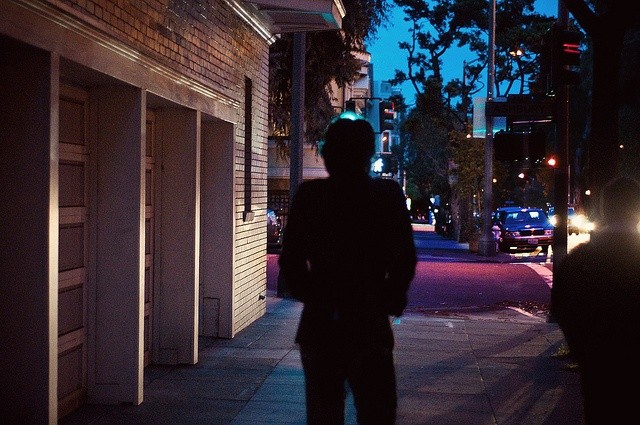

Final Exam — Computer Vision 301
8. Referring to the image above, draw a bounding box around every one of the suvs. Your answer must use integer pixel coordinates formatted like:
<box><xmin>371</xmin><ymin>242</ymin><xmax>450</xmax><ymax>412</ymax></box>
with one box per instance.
<box><xmin>548</xmin><ymin>206</ymin><xmax>580</xmax><ymax>233</ymax></box>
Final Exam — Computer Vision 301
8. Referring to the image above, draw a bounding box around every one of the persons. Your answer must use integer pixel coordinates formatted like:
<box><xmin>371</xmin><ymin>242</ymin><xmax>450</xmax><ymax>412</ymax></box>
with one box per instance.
<box><xmin>276</xmin><ymin>111</ymin><xmax>417</xmax><ymax>425</ymax></box>
<box><xmin>548</xmin><ymin>179</ymin><xmax>640</xmax><ymax>424</ymax></box>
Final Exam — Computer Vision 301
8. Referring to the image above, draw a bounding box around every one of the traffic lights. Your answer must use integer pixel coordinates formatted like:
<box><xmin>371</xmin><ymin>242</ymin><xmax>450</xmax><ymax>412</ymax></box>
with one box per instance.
<box><xmin>373</xmin><ymin>157</ymin><xmax>387</xmax><ymax>172</ymax></box>
<box><xmin>383</xmin><ymin>132</ymin><xmax>389</xmax><ymax>152</ymax></box>
<box><xmin>547</xmin><ymin>154</ymin><xmax>564</xmax><ymax>170</ymax></box>
<box><xmin>557</xmin><ymin>28</ymin><xmax>582</xmax><ymax>63</ymax></box>
<box><xmin>379</xmin><ymin>101</ymin><xmax>398</xmax><ymax>131</ymax></box>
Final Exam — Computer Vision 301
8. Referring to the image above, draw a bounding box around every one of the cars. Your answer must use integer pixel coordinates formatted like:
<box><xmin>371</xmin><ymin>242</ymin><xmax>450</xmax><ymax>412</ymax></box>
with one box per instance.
<box><xmin>494</xmin><ymin>205</ymin><xmax>555</xmax><ymax>248</ymax></box>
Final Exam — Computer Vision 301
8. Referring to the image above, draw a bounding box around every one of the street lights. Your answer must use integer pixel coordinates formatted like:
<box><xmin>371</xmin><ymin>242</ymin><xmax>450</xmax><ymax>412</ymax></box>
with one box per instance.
<box><xmin>584</xmin><ymin>188</ymin><xmax>592</xmax><ymax>213</ymax></box>
<box><xmin>493</xmin><ymin>178</ymin><xmax>501</xmax><ymax>203</ymax></box>
<box><xmin>519</xmin><ymin>171</ymin><xmax>534</xmax><ymax>206</ymax></box>
<box><xmin>545</xmin><ymin>88</ymin><xmax>570</xmax><ymax>324</ymax></box>
<box><xmin>460</xmin><ymin>48</ymin><xmax>524</xmax><ymax>140</ymax></box>
